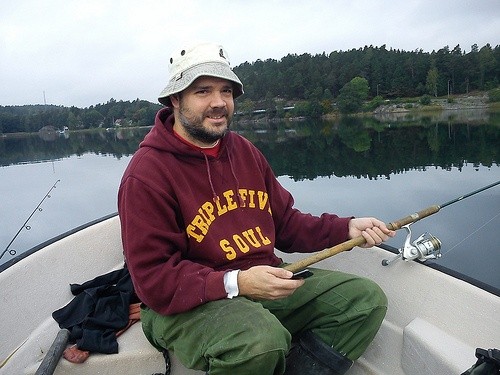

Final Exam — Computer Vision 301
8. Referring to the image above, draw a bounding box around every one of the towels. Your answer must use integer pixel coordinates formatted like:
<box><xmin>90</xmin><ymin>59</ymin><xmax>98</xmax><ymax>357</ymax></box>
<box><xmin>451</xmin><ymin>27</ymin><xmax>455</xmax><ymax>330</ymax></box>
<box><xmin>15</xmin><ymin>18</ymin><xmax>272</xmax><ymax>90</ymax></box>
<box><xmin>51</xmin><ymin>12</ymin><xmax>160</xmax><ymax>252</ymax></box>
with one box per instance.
<box><xmin>52</xmin><ymin>268</ymin><xmax>142</xmax><ymax>355</ymax></box>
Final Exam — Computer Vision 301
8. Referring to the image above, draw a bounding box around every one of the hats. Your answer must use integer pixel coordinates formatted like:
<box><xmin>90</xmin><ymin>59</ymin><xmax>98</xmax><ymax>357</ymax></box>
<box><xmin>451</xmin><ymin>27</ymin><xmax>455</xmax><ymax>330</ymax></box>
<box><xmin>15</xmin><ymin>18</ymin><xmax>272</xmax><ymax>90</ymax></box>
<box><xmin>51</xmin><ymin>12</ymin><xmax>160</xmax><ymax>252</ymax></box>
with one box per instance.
<box><xmin>157</xmin><ymin>36</ymin><xmax>244</xmax><ymax>108</ymax></box>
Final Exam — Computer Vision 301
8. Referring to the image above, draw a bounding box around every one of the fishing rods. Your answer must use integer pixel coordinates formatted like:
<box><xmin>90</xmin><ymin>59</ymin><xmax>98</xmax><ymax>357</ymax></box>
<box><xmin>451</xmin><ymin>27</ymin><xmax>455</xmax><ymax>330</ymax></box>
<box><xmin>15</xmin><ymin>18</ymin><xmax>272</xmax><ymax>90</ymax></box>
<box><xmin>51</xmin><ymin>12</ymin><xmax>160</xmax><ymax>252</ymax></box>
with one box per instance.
<box><xmin>1</xmin><ymin>179</ymin><xmax>60</xmax><ymax>257</ymax></box>
<box><xmin>282</xmin><ymin>180</ymin><xmax>500</xmax><ymax>275</ymax></box>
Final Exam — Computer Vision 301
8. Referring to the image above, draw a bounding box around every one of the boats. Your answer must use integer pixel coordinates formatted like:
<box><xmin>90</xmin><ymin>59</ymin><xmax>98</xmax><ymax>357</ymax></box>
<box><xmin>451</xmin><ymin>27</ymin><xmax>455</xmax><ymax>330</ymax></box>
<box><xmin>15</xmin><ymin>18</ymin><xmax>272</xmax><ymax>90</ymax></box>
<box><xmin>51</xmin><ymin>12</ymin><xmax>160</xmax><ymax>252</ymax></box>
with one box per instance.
<box><xmin>0</xmin><ymin>209</ymin><xmax>499</xmax><ymax>372</ymax></box>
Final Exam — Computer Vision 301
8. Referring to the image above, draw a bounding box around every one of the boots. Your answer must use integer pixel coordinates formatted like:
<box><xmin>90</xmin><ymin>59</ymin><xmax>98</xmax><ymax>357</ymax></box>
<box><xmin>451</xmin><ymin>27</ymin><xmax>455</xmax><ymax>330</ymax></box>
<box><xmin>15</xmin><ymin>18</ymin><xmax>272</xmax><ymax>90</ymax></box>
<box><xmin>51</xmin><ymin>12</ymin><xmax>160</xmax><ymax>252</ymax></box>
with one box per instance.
<box><xmin>284</xmin><ymin>330</ymin><xmax>352</xmax><ymax>374</ymax></box>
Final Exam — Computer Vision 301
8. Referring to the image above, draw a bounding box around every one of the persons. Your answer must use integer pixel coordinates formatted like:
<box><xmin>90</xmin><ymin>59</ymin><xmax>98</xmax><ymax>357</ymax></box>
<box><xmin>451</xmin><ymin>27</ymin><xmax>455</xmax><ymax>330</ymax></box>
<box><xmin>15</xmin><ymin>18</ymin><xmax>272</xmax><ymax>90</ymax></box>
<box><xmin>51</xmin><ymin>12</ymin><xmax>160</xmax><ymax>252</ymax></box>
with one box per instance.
<box><xmin>118</xmin><ymin>44</ymin><xmax>395</xmax><ymax>374</ymax></box>
